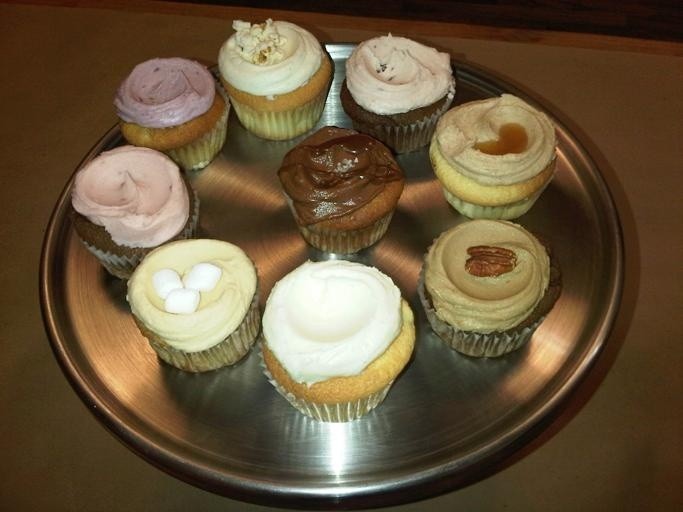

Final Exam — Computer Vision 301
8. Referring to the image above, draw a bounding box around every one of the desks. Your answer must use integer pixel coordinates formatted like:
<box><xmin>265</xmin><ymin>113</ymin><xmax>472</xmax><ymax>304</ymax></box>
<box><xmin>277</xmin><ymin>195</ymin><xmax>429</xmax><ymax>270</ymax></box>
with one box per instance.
<box><xmin>0</xmin><ymin>0</ymin><xmax>681</xmax><ymax>510</ymax></box>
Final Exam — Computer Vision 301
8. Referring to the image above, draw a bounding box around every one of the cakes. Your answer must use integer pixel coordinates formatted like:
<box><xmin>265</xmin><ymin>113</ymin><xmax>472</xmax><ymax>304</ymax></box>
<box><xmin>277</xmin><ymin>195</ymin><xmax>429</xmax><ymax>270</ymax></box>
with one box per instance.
<box><xmin>417</xmin><ymin>221</ymin><xmax>562</xmax><ymax>358</ymax></box>
<box><xmin>429</xmin><ymin>94</ymin><xmax>560</xmax><ymax>220</ymax></box>
<box><xmin>276</xmin><ymin>125</ymin><xmax>405</xmax><ymax>254</ymax></box>
<box><xmin>340</xmin><ymin>33</ymin><xmax>456</xmax><ymax>155</ymax></box>
<box><xmin>127</xmin><ymin>237</ymin><xmax>262</xmax><ymax>374</ymax></box>
<box><xmin>216</xmin><ymin>18</ymin><xmax>334</xmax><ymax>141</ymax></box>
<box><xmin>262</xmin><ymin>257</ymin><xmax>416</xmax><ymax>420</ymax></box>
<box><xmin>113</xmin><ymin>58</ymin><xmax>231</xmax><ymax>172</ymax></box>
<box><xmin>69</xmin><ymin>146</ymin><xmax>201</xmax><ymax>280</ymax></box>
